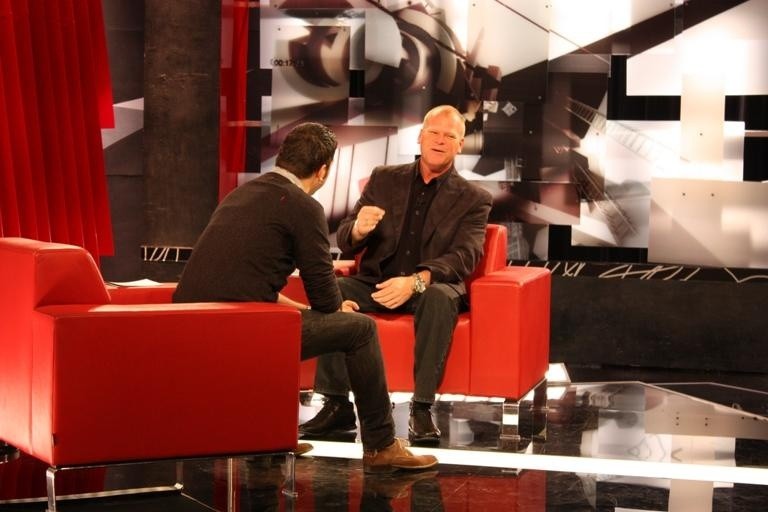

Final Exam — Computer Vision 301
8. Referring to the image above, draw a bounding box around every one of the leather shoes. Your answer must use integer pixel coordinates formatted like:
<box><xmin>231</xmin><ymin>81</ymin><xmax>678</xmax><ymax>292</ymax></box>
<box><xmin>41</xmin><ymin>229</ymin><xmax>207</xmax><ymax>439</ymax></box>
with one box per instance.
<box><xmin>298</xmin><ymin>400</ymin><xmax>357</xmax><ymax>434</ymax></box>
<box><xmin>295</xmin><ymin>443</ymin><xmax>314</xmax><ymax>455</ymax></box>
<box><xmin>408</xmin><ymin>407</ymin><xmax>441</xmax><ymax>442</ymax></box>
<box><xmin>364</xmin><ymin>438</ymin><xmax>439</xmax><ymax>474</ymax></box>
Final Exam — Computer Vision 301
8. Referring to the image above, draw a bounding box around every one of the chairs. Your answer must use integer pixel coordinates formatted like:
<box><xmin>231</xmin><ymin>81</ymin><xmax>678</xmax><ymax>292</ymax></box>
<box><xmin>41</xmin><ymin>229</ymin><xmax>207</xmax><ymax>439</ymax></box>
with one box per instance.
<box><xmin>0</xmin><ymin>236</ymin><xmax>303</xmax><ymax>512</ymax></box>
<box><xmin>281</xmin><ymin>224</ymin><xmax>552</xmax><ymax>440</ymax></box>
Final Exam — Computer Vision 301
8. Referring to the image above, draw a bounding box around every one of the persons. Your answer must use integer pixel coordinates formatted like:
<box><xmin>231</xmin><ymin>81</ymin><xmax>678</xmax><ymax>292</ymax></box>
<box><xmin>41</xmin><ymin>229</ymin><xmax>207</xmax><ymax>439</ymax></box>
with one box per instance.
<box><xmin>244</xmin><ymin>457</ymin><xmax>447</xmax><ymax>512</ymax></box>
<box><xmin>170</xmin><ymin>122</ymin><xmax>440</xmax><ymax>475</ymax></box>
<box><xmin>299</xmin><ymin>104</ymin><xmax>493</xmax><ymax>443</ymax></box>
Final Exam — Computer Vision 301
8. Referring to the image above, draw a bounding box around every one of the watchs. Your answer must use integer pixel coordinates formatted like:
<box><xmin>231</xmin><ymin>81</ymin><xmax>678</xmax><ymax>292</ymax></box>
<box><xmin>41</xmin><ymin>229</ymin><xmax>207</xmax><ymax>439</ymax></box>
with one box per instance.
<box><xmin>410</xmin><ymin>272</ymin><xmax>426</xmax><ymax>295</ymax></box>
